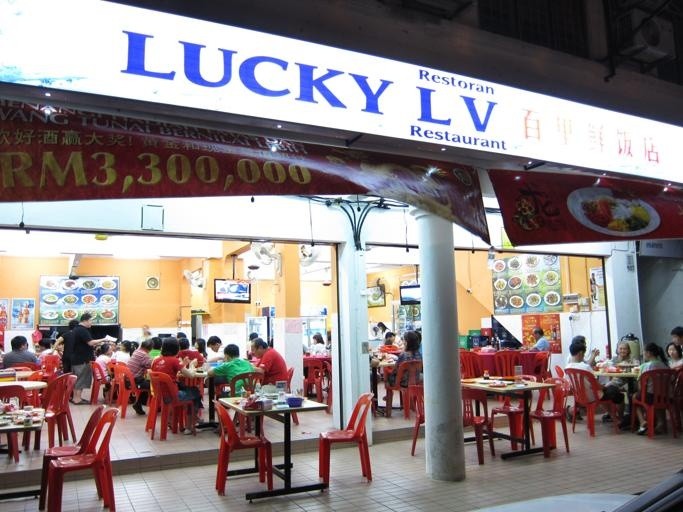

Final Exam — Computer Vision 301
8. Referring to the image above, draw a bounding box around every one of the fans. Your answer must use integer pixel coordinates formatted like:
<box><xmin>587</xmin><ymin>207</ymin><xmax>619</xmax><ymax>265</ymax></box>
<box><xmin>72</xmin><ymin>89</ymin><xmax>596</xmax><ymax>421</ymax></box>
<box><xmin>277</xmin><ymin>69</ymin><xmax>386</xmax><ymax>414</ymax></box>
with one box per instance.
<box><xmin>250</xmin><ymin>240</ymin><xmax>283</xmax><ymax>276</ymax></box>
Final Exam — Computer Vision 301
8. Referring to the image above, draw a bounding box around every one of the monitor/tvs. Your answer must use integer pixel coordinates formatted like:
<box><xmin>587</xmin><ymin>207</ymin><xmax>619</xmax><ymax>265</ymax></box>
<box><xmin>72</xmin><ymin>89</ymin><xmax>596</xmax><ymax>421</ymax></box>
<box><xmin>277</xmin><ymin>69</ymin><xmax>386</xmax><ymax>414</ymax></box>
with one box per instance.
<box><xmin>400</xmin><ymin>285</ymin><xmax>420</xmax><ymax>305</ymax></box>
<box><xmin>214</xmin><ymin>279</ymin><xmax>251</xmax><ymax>303</ymax></box>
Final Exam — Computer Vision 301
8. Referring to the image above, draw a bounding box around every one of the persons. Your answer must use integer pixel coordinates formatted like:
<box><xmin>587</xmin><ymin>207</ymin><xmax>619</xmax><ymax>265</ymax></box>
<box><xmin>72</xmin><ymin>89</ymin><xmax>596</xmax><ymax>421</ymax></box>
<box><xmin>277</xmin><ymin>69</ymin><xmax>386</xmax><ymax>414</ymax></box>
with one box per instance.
<box><xmin>302</xmin><ymin>322</ymin><xmax>422</xmax><ymax>416</ymax></box>
<box><xmin>1</xmin><ymin>313</ymin><xmax>289</xmax><ymax>435</ymax></box>
<box><xmin>528</xmin><ymin>326</ymin><xmax>682</xmax><ymax>437</ymax></box>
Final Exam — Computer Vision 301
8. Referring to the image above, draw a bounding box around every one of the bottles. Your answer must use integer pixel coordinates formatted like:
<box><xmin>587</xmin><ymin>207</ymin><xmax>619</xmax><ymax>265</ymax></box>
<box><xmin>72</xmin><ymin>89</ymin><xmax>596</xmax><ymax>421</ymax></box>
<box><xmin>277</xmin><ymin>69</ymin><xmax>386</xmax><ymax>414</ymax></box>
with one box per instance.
<box><xmin>483</xmin><ymin>369</ymin><xmax>489</xmax><ymax>380</ymax></box>
<box><xmin>255</xmin><ymin>300</ymin><xmax>277</xmax><ymax>316</ymax></box>
<box><xmin>240</xmin><ymin>390</ymin><xmax>247</xmax><ymax>407</ymax></box>
<box><xmin>18</xmin><ymin>303</ymin><xmax>29</xmax><ymax>324</ymax></box>
<box><xmin>491</xmin><ymin>335</ymin><xmax>499</xmax><ymax>350</ymax></box>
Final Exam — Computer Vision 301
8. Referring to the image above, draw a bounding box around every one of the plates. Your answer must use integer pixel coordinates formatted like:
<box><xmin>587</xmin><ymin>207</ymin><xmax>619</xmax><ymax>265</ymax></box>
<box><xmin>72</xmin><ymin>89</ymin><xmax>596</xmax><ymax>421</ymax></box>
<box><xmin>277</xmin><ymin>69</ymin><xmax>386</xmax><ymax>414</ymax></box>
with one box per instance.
<box><xmin>0</xmin><ymin>404</ymin><xmax>45</xmax><ymax>426</ymax></box>
<box><xmin>493</xmin><ymin>255</ymin><xmax>559</xmax><ymax>308</ymax></box>
<box><xmin>40</xmin><ymin>279</ymin><xmax>116</xmax><ymax>320</ymax></box>
<box><xmin>478</xmin><ymin>380</ymin><xmax>512</xmax><ymax>385</ymax></box>
<box><xmin>566</xmin><ymin>186</ymin><xmax>661</xmax><ymax>236</ymax></box>
<box><xmin>615</xmin><ymin>364</ymin><xmax>639</xmax><ymax>368</ymax></box>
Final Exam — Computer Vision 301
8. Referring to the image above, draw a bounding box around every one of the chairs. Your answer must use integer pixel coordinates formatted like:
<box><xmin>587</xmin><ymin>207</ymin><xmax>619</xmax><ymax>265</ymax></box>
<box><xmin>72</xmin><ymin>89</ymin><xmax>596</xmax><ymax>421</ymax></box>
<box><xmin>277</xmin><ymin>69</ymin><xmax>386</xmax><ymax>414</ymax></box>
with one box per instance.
<box><xmin>1</xmin><ymin>349</ymin><xmax>81</xmax><ymax>449</ymax></box>
<box><xmin>211</xmin><ymin>399</ymin><xmax>274</xmax><ymax>498</ymax></box>
<box><xmin>47</xmin><ymin>407</ymin><xmax>120</xmax><ymax>511</ymax></box>
<box><xmin>106</xmin><ymin>348</ymin><xmax>332</xmax><ymax>440</ymax></box>
<box><xmin>317</xmin><ymin>391</ymin><xmax>375</xmax><ymax>488</ymax></box>
<box><xmin>39</xmin><ymin>402</ymin><xmax>105</xmax><ymax>511</ymax></box>
<box><xmin>379</xmin><ymin>352</ymin><xmax>681</xmax><ymax>464</ymax></box>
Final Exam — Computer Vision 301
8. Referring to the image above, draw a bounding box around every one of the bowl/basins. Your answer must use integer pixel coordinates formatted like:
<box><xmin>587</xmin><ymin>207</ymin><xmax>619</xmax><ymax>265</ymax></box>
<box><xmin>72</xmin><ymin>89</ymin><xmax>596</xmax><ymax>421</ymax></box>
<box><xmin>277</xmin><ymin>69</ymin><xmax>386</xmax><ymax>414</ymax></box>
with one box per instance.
<box><xmin>288</xmin><ymin>397</ymin><xmax>303</xmax><ymax>406</ymax></box>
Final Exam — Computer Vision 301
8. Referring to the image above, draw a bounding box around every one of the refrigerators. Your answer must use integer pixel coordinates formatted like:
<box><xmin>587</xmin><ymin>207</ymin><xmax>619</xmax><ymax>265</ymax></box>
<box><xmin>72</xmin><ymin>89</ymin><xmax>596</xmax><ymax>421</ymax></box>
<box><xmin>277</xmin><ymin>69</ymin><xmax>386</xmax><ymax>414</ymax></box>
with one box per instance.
<box><xmin>244</xmin><ymin>316</ymin><xmax>305</xmax><ymax>396</ymax></box>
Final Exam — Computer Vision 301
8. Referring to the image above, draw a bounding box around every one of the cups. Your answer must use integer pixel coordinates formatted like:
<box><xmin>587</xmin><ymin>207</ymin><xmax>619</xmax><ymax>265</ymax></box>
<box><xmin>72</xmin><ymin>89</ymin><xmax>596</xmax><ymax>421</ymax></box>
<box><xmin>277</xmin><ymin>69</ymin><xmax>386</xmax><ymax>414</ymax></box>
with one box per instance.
<box><xmin>196</xmin><ymin>367</ymin><xmax>203</xmax><ymax>373</ymax></box>
<box><xmin>513</xmin><ymin>365</ymin><xmax>522</xmax><ymax>383</ymax></box>
<box><xmin>277</xmin><ymin>391</ymin><xmax>285</xmax><ymax>406</ymax></box>
<box><xmin>290</xmin><ymin>388</ymin><xmax>297</xmax><ymax>397</ymax></box>
<box><xmin>381</xmin><ymin>352</ymin><xmax>387</xmax><ymax>361</ymax></box>
<box><xmin>473</xmin><ymin>338</ymin><xmax>478</xmax><ymax>347</ymax></box>
